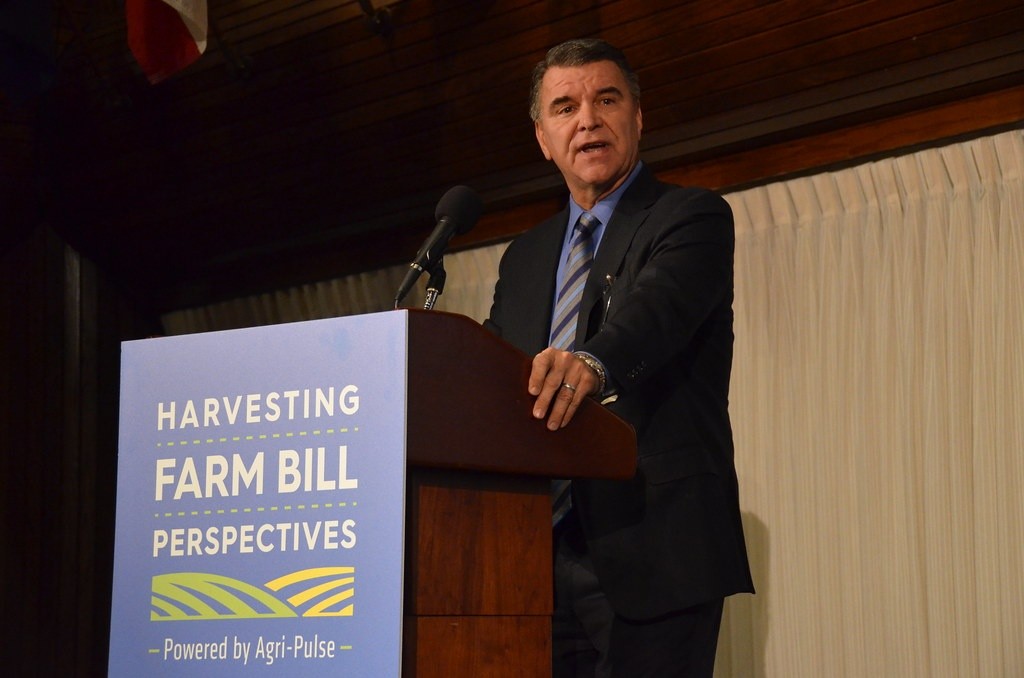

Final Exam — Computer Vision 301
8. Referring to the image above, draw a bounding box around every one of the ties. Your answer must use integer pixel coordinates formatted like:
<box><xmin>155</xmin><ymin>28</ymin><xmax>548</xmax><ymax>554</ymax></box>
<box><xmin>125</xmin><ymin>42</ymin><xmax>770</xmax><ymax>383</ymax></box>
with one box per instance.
<box><xmin>549</xmin><ymin>212</ymin><xmax>600</xmax><ymax>354</ymax></box>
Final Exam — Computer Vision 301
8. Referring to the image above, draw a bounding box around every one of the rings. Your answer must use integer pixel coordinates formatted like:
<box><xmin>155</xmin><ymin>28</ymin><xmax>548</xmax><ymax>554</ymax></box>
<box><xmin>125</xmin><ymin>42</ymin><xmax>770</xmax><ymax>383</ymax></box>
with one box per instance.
<box><xmin>562</xmin><ymin>383</ymin><xmax>576</xmax><ymax>390</ymax></box>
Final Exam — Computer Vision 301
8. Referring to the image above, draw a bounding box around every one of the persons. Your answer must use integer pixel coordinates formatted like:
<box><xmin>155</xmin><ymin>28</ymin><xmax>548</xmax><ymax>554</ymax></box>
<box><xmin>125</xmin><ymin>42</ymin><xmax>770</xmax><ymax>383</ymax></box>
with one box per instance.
<box><xmin>472</xmin><ymin>38</ymin><xmax>756</xmax><ymax>678</ymax></box>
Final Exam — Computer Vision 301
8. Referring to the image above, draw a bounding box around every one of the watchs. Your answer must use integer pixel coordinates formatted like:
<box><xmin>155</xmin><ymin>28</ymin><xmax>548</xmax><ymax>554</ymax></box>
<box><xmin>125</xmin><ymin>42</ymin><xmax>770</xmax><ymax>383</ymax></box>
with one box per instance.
<box><xmin>576</xmin><ymin>353</ymin><xmax>606</xmax><ymax>394</ymax></box>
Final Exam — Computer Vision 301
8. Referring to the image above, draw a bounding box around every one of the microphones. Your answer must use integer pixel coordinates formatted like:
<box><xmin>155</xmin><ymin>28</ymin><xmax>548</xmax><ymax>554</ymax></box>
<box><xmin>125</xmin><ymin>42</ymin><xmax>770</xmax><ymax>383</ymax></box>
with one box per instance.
<box><xmin>394</xmin><ymin>186</ymin><xmax>486</xmax><ymax>301</ymax></box>
